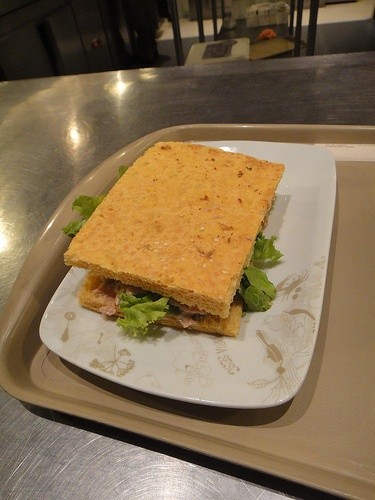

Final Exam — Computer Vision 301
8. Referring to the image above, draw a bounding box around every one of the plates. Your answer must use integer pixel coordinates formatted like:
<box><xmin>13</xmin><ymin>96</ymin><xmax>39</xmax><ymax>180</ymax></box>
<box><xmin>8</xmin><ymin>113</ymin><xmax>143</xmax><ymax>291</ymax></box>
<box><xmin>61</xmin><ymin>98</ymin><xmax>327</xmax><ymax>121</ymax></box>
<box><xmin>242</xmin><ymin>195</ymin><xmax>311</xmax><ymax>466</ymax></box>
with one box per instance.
<box><xmin>39</xmin><ymin>142</ymin><xmax>337</xmax><ymax>408</ymax></box>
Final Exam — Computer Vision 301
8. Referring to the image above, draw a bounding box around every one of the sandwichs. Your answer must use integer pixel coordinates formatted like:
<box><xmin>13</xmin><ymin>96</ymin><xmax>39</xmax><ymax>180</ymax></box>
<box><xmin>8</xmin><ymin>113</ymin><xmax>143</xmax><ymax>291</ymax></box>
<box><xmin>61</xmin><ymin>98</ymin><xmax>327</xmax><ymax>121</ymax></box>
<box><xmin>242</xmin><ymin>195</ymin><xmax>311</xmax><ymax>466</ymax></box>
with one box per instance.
<box><xmin>61</xmin><ymin>142</ymin><xmax>285</xmax><ymax>338</ymax></box>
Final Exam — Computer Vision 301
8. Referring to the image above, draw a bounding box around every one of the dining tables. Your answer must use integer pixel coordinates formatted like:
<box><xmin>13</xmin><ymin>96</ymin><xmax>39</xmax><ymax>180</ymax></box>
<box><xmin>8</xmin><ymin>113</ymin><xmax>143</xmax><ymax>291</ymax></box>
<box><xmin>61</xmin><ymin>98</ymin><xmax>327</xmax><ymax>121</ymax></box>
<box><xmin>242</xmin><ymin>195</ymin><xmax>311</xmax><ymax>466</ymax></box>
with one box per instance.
<box><xmin>0</xmin><ymin>50</ymin><xmax>374</xmax><ymax>500</ymax></box>
